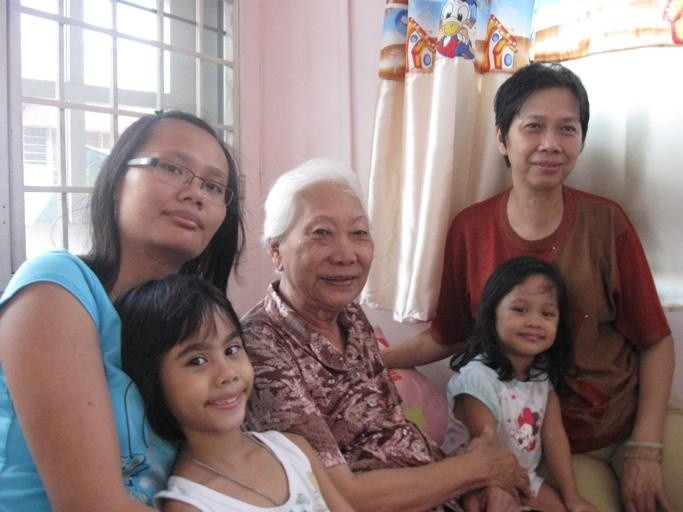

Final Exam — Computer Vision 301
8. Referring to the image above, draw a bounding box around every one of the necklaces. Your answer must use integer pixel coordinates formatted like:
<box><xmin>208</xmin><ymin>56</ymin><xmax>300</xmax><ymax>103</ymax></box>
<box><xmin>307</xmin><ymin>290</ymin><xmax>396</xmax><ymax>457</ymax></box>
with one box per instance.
<box><xmin>180</xmin><ymin>428</ymin><xmax>287</xmax><ymax>507</ymax></box>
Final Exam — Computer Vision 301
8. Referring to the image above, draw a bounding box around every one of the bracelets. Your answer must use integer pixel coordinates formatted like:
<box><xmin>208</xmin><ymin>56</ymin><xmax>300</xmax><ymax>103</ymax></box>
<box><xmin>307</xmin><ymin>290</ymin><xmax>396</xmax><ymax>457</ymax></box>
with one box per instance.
<box><xmin>624</xmin><ymin>452</ymin><xmax>662</xmax><ymax>461</ymax></box>
<box><xmin>626</xmin><ymin>440</ymin><xmax>663</xmax><ymax>449</ymax></box>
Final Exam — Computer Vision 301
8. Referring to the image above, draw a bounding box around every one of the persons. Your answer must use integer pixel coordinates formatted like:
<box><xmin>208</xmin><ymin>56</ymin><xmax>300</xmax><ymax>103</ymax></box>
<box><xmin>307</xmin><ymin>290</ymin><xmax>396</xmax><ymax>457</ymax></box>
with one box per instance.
<box><xmin>441</xmin><ymin>256</ymin><xmax>598</xmax><ymax>512</ymax></box>
<box><xmin>235</xmin><ymin>156</ymin><xmax>532</xmax><ymax>512</ymax></box>
<box><xmin>0</xmin><ymin>109</ymin><xmax>247</xmax><ymax>512</ymax></box>
<box><xmin>377</xmin><ymin>61</ymin><xmax>675</xmax><ymax>511</ymax></box>
<box><xmin>114</xmin><ymin>273</ymin><xmax>357</xmax><ymax>512</ymax></box>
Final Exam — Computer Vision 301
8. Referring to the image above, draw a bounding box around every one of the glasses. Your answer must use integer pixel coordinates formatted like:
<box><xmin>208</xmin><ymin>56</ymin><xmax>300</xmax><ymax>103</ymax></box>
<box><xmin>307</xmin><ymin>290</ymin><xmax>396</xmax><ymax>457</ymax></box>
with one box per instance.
<box><xmin>126</xmin><ymin>157</ymin><xmax>234</xmax><ymax>207</ymax></box>
